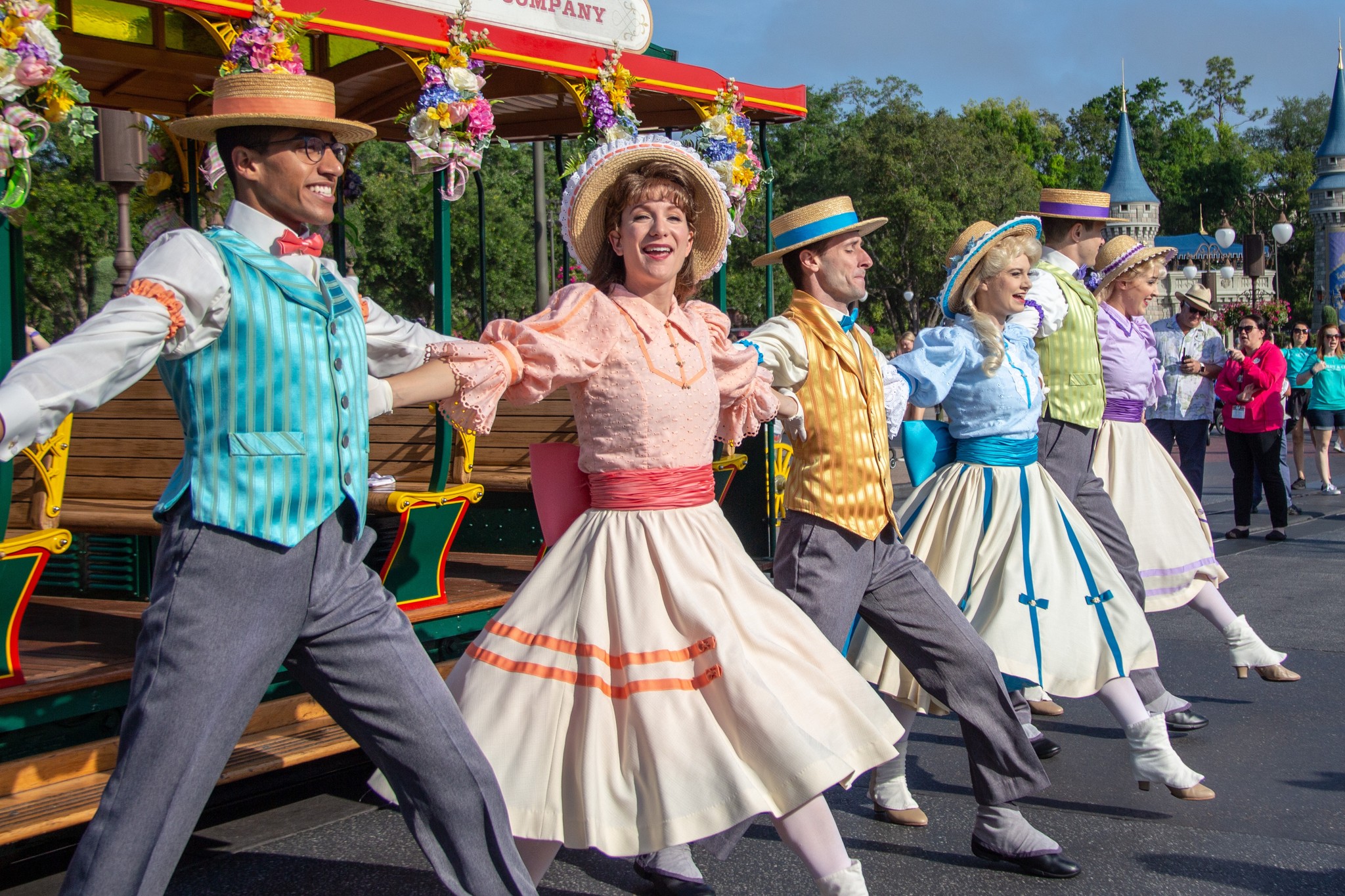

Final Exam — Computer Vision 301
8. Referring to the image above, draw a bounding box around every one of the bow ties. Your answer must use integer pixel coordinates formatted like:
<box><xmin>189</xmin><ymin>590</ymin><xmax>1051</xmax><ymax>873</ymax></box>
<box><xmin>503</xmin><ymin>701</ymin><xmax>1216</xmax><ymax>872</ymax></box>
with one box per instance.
<box><xmin>840</xmin><ymin>308</ymin><xmax>858</xmax><ymax>334</ymax></box>
<box><xmin>276</xmin><ymin>229</ymin><xmax>324</xmax><ymax>257</ymax></box>
<box><xmin>1077</xmin><ymin>263</ymin><xmax>1087</xmax><ymax>280</ymax></box>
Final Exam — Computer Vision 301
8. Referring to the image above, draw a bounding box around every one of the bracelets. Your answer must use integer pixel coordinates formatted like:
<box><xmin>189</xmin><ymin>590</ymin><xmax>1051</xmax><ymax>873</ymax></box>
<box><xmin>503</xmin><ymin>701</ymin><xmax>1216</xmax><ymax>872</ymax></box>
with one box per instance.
<box><xmin>1310</xmin><ymin>367</ymin><xmax>1316</xmax><ymax>376</ymax></box>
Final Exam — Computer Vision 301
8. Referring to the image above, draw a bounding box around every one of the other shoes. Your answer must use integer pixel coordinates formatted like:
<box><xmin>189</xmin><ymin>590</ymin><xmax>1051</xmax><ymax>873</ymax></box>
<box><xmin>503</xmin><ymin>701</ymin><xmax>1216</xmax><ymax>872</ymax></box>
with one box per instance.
<box><xmin>1321</xmin><ymin>479</ymin><xmax>1332</xmax><ymax>491</ymax></box>
<box><xmin>1322</xmin><ymin>484</ymin><xmax>1341</xmax><ymax>495</ymax></box>
<box><xmin>1287</xmin><ymin>504</ymin><xmax>1303</xmax><ymax>516</ymax></box>
<box><xmin>1334</xmin><ymin>441</ymin><xmax>1345</xmax><ymax>452</ymax></box>
<box><xmin>1291</xmin><ymin>477</ymin><xmax>1306</xmax><ymax>490</ymax></box>
<box><xmin>897</xmin><ymin>456</ymin><xmax>905</xmax><ymax>462</ymax></box>
<box><xmin>1265</xmin><ymin>530</ymin><xmax>1287</xmax><ymax>541</ymax></box>
<box><xmin>1225</xmin><ymin>527</ymin><xmax>1249</xmax><ymax>539</ymax></box>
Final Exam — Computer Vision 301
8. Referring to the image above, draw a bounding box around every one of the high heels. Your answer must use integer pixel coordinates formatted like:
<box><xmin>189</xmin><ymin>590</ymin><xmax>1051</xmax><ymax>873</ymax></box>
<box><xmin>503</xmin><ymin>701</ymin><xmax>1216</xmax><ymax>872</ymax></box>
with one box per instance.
<box><xmin>1139</xmin><ymin>778</ymin><xmax>1216</xmax><ymax>801</ymax></box>
<box><xmin>874</xmin><ymin>800</ymin><xmax>929</xmax><ymax>826</ymax></box>
<box><xmin>1026</xmin><ymin>700</ymin><xmax>1063</xmax><ymax>715</ymax></box>
<box><xmin>1236</xmin><ymin>665</ymin><xmax>1300</xmax><ymax>683</ymax></box>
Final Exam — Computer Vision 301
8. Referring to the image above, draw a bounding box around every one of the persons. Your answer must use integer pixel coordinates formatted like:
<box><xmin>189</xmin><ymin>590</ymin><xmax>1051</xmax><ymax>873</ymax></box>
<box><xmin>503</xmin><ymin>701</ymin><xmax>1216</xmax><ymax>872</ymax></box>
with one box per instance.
<box><xmin>1248</xmin><ymin>322</ymin><xmax>1345</xmax><ymax>514</ymax></box>
<box><xmin>846</xmin><ymin>215</ymin><xmax>1215</xmax><ymax>826</ymax></box>
<box><xmin>889</xmin><ymin>187</ymin><xmax>1302</xmax><ymax>757</ymax></box>
<box><xmin>732</xmin><ymin>196</ymin><xmax>1081</xmax><ymax>896</ymax></box>
<box><xmin>0</xmin><ymin>70</ymin><xmax>543</xmax><ymax>896</ymax></box>
<box><xmin>369</xmin><ymin>136</ymin><xmax>871</xmax><ymax>896</ymax></box>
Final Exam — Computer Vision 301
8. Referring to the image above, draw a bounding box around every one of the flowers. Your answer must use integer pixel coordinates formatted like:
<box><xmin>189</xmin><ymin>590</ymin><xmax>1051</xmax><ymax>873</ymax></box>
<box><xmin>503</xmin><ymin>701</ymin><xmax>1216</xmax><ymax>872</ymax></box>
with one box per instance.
<box><xmin>556</xmin><ymin>38</ymin><xmax>646</xmax><ymax>180</ymax></box>
<box><xmin>1206</xmin><ymin>297</ymin><xmax>1291</xmax><ymax>337</ymax></box>
<box><xmin>189</xmin><ymin>0</ymin><xmax>327</xmax><ymax>101</ymax></box>
<box><xmin>394</xmin><ymin>0</ymin><xmax>505</xmax><ymax>202</ymax></box>
<box><xmin>122</xmin><ymin>118</ymin><xmax>225</xmax><ymax>239</ymax></box>
<box><xmin>0</xmin><ymin>0</ymin><xmax>101</xmax><ymax>240</ymax></box>
<box><xmin>310</xmin><ymin>169</ymin><xmax>364</xmax><ymax>262</ymax></box>
<box><xmin>682</xmin><ymin>76</ymin><xmax>766</xmax><ymax>241</ymax></box>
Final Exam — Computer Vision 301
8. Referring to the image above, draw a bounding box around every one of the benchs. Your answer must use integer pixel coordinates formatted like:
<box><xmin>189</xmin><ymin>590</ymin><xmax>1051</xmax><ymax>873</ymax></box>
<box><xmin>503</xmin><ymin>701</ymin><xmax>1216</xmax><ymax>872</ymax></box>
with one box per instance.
<box><xmin>7</xmin><ymin>366</ymin><xmax>580</xmax><ymax>542</ymax></box>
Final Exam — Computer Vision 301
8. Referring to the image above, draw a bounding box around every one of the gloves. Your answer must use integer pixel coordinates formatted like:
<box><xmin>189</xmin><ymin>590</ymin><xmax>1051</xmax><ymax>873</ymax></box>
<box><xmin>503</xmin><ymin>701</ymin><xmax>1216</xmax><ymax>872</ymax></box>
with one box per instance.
<box><xmin>367</xmin><ymin>374</ymin><xmax>393</xmax><ymax>419</ymax></box>
<box><xmin>777</xmin><ymin>386</ymin><xmax>808</xmax><ymax>443</ymax></box>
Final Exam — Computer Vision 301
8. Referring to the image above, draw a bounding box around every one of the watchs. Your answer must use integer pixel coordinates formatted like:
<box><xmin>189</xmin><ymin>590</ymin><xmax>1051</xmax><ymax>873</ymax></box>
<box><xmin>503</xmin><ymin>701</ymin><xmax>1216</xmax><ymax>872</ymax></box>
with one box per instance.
<box><xmin>1198</xmin><ymin>362</ymin><xmax>1205</xmax><ymax>374</ymax></box>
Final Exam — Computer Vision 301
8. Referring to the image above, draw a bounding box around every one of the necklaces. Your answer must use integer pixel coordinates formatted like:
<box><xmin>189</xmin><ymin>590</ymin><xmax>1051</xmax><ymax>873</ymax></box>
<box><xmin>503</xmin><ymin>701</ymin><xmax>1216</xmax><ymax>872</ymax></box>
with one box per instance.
<box><xmin>1295</xmin><ymin>345</ymin><xmax>1306</xmax><ymax>352</ymax></box>
<box><xmin>1325</xmin><ymin>354</ymin><xmax>1335</xmax><ymax>364</ymax></box>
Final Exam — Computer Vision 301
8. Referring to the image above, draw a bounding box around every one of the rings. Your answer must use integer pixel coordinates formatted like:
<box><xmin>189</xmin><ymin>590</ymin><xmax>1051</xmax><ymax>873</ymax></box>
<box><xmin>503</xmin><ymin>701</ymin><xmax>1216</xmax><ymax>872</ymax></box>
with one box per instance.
<box><xmin>1188</xmin><ymin>367</ymin><xmax>1189</xmax><ymax>371</ymax></box>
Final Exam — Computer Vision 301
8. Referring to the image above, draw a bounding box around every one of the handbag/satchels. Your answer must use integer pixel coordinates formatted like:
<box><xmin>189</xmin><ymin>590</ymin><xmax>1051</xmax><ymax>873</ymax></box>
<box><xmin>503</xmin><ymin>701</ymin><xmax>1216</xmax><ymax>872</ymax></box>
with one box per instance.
<box><xmin>1285</xmin><ymin>406</ymin><xmax>1297</xmax><ymax>435</ymax></box>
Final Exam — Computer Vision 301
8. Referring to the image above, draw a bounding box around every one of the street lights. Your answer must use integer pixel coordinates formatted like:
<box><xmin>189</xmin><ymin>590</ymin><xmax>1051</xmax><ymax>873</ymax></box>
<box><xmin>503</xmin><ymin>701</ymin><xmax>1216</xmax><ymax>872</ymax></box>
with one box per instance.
<box><xmin>1183</xmin><ymin>243</ymin><xmax>1234</xmax><ymax>326</ymax></box>
<box><xmin>1217</xmin><ymin>191</ymin><xmax>1293</xmax><ymax>311</ymax></box>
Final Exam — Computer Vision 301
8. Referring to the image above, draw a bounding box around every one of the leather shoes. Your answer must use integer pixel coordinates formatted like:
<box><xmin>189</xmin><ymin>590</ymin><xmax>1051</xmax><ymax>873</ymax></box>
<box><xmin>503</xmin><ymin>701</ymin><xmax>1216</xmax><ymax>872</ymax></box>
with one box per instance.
<box><xmin>632</xmin><ymin>859</ymin><xmax>715</xmax><ymax>896</ymax></box>
<box><xmin>1031</xmin><ymin>737</ymin><xmax>1060</xmax><ymax>758</ymax></box>
<box><xmin>1165</xmin><ymin>712</ymin><xmax>1209</xmax><ymax>731</ymax></box>
<box><xmin>970</xmin><ymin>832</ymin><xmax>1081</xmax><ymax>878</ymax></box>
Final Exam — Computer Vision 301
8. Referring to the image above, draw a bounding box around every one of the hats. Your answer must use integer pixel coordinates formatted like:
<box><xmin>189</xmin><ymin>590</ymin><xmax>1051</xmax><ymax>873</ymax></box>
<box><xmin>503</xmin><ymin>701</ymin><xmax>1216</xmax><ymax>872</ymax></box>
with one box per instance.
<box><xmin>752</xmin><ymin>195</ymin><xmax>888</xmax><ymax>267</ymax></box>
<box><xmin>1017</xmin><ymin>188</ymin><xmax>1129</xmax><ymax>222</ymax></box>
<box><xmin>937</xmin><ymin>214</ymin><xmax>1042</xmax><ymax>326</ymax></box>
<box><xmin>1175</xmin><ymin>284</ymin><xmax>1217</xmax><ymax>312</ymax></box>
<box><xmin>559</xmin><ymin>135</ymin><xmax>735</xmax><ymax>285</ymax></box>
<box><xmin>737</xmin><ymin>331</ymin><xmax>750</xmax><ymax>337</ymax></box>
<box><xmin>169</xmin><ymin>73</ymin><xmax>376</xmax><ymax>143</ymax></box>
<box><xmin>1085</xmin><ymin>235</ymin><xmax>1178</xmax><ymax>297</ymax></box>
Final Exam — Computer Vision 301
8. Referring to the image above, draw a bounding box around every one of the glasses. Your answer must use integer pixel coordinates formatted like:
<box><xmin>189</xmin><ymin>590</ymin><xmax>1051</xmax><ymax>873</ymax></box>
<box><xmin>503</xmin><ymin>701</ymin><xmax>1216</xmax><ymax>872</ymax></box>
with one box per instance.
<box><xmin>1325</xmin><ymin>334</ymin><xmax>1341</xmax><ymax>340</ymax></box>
<box><xmin>252</xmin><ymin>136</ymin><xmax>347</xmax><ymax>166</ymax></box>
<box><xmin>1236</xmin><ymin>326</ymin><xmax>1260</xmax><ymax>334</ymax></box>
<box><xmin>1293</xmin><ymin>328</ymin><xmax>1309</xmax><ymax>334</ymax></box>
<box><xmin>1184</xmin><ymin>301</ymin><xmax>1208</xmax><ymax>316</ymax></box>
